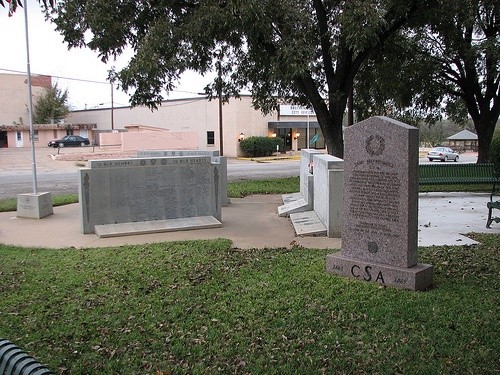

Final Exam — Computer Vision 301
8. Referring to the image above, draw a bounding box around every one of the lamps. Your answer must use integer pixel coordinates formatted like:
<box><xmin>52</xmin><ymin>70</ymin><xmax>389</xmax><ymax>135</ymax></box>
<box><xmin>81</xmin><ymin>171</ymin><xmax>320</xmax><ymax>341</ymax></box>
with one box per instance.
<box><xmin>238</xmin><ymin>132</ymin><xmax>245</xmax><ymax>142</ymax></box>
<box><xmin>272</xmin><ymin>131</ymin><xmax>277</xmax><ymax>137</ymax></box>
<box><xmin>294</xmin><ymin>131</ymin><xmax>301</xmax><ymax>140</ymax></box>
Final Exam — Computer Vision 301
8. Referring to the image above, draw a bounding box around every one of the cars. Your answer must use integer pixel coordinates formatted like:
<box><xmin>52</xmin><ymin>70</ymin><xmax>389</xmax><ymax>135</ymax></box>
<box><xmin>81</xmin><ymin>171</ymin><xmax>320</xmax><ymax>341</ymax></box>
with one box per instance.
<box><xmin>427</xmin><ymin>146</ymin><xmax>460</xmax><ymax>162</ymax></box>
<box><xmin>48</xmin><ymin>134</ymin><xmax>90</xmax><ymax>147</ymax></box>
